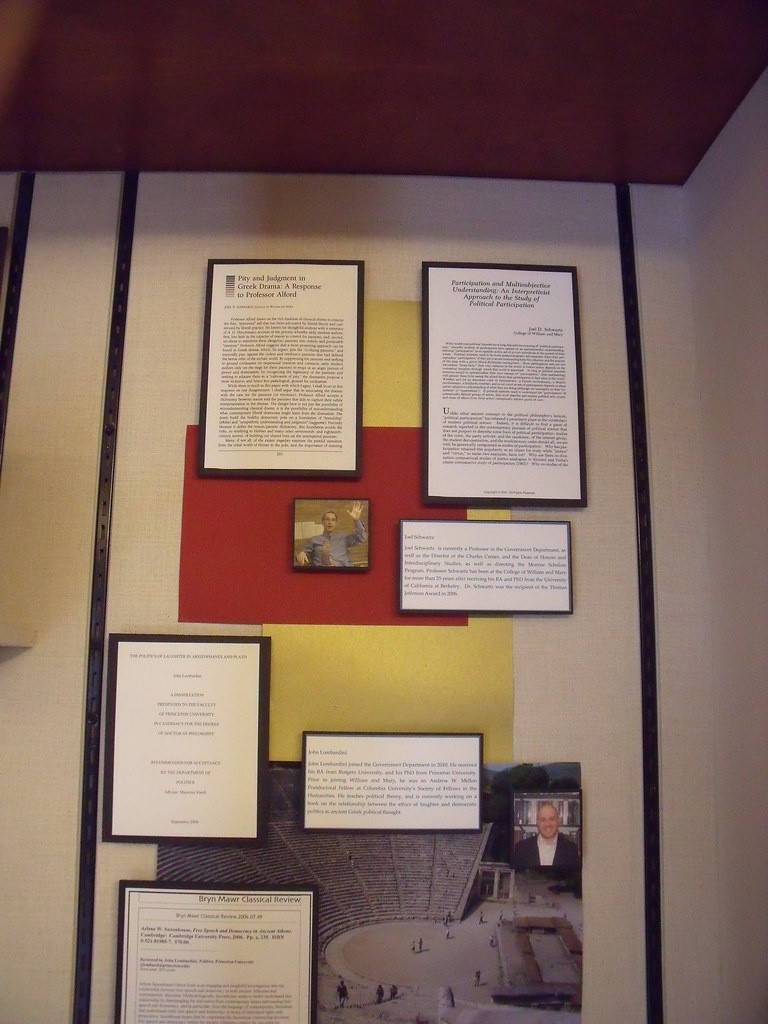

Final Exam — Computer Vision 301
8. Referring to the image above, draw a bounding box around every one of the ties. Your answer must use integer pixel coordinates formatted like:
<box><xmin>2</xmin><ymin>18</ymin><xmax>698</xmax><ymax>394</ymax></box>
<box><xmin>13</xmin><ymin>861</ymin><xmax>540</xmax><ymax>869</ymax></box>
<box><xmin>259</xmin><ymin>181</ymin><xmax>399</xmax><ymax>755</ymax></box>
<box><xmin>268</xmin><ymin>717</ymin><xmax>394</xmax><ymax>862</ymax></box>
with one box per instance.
<box><xmin>322</xmin><ymin>535</ymin><xmax>330</xmax><ymax>567</ymax></box>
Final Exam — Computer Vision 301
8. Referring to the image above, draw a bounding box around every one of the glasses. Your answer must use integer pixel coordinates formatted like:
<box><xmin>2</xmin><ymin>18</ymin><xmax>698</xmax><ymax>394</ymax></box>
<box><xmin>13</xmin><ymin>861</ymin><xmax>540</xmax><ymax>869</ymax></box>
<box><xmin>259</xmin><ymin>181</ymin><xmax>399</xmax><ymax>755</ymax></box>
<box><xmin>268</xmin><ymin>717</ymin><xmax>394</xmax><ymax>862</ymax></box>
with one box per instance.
<box><xmin>323</xmin><ymin>518</ymin><xmax>336</xmax><ymax>521</ymax></box>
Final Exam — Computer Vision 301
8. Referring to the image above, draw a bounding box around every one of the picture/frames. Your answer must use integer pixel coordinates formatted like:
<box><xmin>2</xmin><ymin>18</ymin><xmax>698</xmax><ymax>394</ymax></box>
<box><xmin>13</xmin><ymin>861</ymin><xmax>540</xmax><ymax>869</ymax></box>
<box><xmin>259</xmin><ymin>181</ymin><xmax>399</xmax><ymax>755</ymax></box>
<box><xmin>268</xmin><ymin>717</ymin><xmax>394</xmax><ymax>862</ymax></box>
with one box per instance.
<box><xmin>116</xmin><ymin>879</ymin><xmax>317</xmax><ymax>1024</ymax></box>
<box><xmin>511</xmin><ymin>789</ymin><xmax>583</xmax><ymax>871</ymax></box>
<box><xmin>423</xmin><ymin>261</ymin><xmax>587</xmax><ymax>507</ymax></box>
<box><xmin>292</xmin><ymin>498</ymin><xmax>370</xmax><ymax>573</ymax></box>
<box><xmin>302</xmin><ymin>732</ymin><xmax>483</xmax><ymax>834</ymax></box>
<box><xmin>198</xmin><ymin>259</ymin><xmax>365</xmax><ymax>481</ymax></box>
<box><xmin>398</xmin><ymin>520</ymin><xmax>572</xmax><ymax>614</ymax></box>
<box><xmin>102</xmin><ymin>632</ymin><xmax>271</xmax><ymax>846</ymax></box>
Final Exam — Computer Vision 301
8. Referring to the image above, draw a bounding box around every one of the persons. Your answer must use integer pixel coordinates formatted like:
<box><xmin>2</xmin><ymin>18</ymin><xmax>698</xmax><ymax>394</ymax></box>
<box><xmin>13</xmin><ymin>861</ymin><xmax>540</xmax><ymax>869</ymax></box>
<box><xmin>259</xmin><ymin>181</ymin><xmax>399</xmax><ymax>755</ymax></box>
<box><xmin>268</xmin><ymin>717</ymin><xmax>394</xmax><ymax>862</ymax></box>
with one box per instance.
<box><xmin>411</xmin><ymin>905</ymin><xmax>520</xmax><ymax>953</ymax></box>
<box><xmin>337</xmin><ymin>980</ymin><xmax>349</xmax><ymax>1008</ymax></box>
<box><xmin>390</xmin><ymin>984</ymin><xmax>398</xmax><ymax>1000</ymax></box>
<box><xmin>297</xmin><ymin>500</ymin><xmax>365</xmax><ymax>567</ymax></box>
<box><xmin>510</xmin><ymin>804</ymin><xmax>578</xmax><ymax>866</ymax></box>
<box><xmin>474</xmin><ymin>970</ymin><xmax>481</xmax><ymax>987</ymax></box>
<box><xmin>375</xmin><ymin>985</ymin><xmax>384</xmax><ymax>1005</ymax></box>
